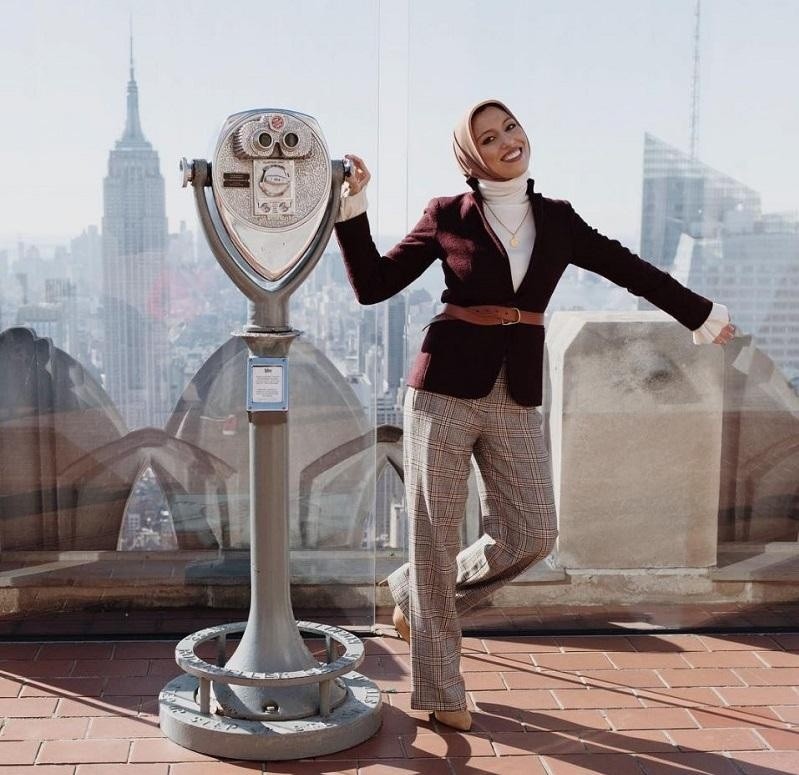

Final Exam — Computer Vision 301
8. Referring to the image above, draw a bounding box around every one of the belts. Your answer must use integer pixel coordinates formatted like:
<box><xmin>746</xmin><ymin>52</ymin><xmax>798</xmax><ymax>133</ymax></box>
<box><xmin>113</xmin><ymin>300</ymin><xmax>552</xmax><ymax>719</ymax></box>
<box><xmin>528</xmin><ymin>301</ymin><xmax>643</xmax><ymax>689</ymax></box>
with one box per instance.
<box><xmin>419</xmin><ymin>302</ymin><xmax>545</xmax><ymax>330</ymax></box>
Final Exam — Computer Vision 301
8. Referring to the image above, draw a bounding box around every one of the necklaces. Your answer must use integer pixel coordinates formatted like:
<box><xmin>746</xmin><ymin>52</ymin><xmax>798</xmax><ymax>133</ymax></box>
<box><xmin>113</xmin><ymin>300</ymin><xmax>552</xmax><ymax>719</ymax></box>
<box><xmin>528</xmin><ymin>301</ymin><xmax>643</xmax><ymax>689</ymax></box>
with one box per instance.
<box><xmin>485</xmin><ymin>200</ymin><xmax>530</xmax><ymax>247</ymax></box>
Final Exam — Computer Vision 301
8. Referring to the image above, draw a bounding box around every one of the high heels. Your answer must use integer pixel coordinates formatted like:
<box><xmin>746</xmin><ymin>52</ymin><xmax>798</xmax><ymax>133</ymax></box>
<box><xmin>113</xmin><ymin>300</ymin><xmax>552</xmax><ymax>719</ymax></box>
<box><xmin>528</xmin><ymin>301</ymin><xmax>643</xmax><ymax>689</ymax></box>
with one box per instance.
<box><xmin>434</xmin><ymin>705</ymin><xmax>473</xmax><ymax>731</ymax></box>
<box><xmin>378</xmin><ymin>578</ymin><xmax>410</xmax><ymax>644</ymax></box>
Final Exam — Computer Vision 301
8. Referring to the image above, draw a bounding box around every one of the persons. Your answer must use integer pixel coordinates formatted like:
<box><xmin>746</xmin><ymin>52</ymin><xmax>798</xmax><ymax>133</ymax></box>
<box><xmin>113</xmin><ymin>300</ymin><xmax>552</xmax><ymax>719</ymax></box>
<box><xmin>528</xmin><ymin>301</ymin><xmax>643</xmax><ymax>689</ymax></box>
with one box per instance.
<box><xmin>333</xmin><ymin>98</ymin><xmax>735</xmax><ymax>732</ymax></box>
